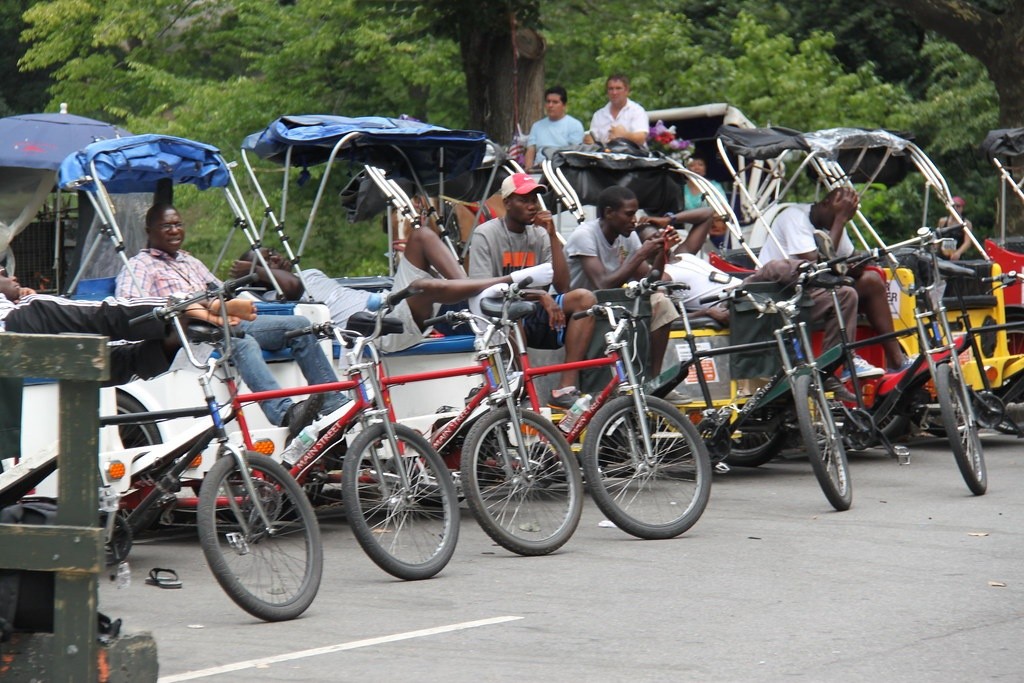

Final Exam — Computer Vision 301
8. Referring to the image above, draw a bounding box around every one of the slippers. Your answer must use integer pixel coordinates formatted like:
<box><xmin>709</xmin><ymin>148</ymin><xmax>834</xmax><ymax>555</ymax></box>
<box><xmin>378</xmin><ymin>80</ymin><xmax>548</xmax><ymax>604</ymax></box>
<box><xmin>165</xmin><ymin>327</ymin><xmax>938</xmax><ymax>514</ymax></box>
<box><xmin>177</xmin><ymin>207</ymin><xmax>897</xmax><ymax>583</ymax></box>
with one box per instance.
<box><xmin>145</xmin><ymin>568</ymin><xmax>182</xmax><ymax>589</ymax></box>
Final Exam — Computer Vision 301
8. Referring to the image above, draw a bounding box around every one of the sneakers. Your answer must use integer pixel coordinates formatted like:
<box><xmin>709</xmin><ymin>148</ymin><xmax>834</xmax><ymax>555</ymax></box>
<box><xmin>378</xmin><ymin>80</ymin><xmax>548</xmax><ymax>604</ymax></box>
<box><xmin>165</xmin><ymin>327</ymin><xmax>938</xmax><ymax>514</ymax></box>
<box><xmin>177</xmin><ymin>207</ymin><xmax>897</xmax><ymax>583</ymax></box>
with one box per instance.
<box><xmin>521</xmin><ymin>395</ymin><xmax>534</xmax><ymax>410</ymax></box>
<box><xmin>663</xmin><ymin>389</ymin><xmax>692</xmax><ymax>404</ymax></box>
<box><xmin>547</xmin><ymin>390</ymin><xmax>582</xmax><ymax>410</ymax></box>
<box><xmin>840</xmin><ymin>354</ymin><xmax>885</xmax><ymax>383</ymax></box>
<box><xmin>288</xmin><ymin>392</ymin><xmax>325</xmax><ymax>440</ymax></box>
<box><xmin>887</xmin><ymin>355</ymin><xmax>916</xmax><ymax>374</ymax></box>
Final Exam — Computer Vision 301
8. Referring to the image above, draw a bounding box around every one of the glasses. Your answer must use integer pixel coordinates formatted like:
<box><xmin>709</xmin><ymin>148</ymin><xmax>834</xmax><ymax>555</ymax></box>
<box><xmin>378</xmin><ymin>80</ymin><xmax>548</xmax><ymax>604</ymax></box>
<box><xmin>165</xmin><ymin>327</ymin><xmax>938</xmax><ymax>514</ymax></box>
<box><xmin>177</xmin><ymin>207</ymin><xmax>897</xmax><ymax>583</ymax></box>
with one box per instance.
<box><xmin>954</xmin><ymin>201</ymin><xmax>961</xmax><ymax>208</ymax></box>
<box><xmin>265</xmin><ymin>248</ymin><xmax>278</xmax><ymax>268</ymax></box>
<box><xmin>148</xmin><ymin>222</ymin><xmax>185</xmax><ymax>230</ymax></box>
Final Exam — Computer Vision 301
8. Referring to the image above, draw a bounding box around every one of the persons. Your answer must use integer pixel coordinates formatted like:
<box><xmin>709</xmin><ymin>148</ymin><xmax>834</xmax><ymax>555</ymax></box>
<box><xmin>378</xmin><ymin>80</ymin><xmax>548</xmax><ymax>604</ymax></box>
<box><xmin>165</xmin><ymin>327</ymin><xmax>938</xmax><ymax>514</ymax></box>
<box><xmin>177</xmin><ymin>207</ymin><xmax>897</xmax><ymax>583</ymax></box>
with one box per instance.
<box><xmin>525</xmin><ymin>83</ymin><xmax>584</xmax><ymax>171</ymax></box>
<box><xmin>113</xmin><ymin>203</ymin><xmax>355</xmax><ymax>435</ymax></box>
<box><xmin>754</xmin><ymin>187</ymin><xmax>921</xmax><ymax>401</ymax></box>
<box><xmin>938</xmin><ymin>196</ymin><xmax>973</xmax><ymax>260</ymax></box>
<box><xmin>585</xmin><ymin>74</ymin><xmax>653</xmax><ymax>187</ymax></box>
<box><xmin>683</xmin><ymin>154</ymin><xmax>729</xmax><ymax>210</ymax></box>
<box><xmin>230</xmin><ymin>225</ymin><xmax>553</xmax><ymax>354</ymax></box>
<box><xmin>0</xmin><ymin>264</ymin><xmax>258</xmax><ymax>387</ymax></box>
<box><xmin>468</xmin><ymin>173</ymin><xmax>599</xmax><ymax>413</ymax></box>
<box><xmin>633</xmin><ymin>207</ymin><xmax>886</xmax><ymax>382</ymax></box>
<box><xmin>561</xmin><ymin>186</ymin><xmax>694</xmax><ymax>405</ymax></box>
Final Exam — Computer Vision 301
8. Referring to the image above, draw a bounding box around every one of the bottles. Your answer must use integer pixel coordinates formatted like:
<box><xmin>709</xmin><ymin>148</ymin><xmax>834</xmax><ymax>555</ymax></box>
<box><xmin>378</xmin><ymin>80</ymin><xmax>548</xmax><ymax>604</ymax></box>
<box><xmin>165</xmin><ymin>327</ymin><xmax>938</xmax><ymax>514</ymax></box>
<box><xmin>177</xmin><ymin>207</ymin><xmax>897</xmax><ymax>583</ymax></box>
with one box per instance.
<box><xmin>119</xmin><ymin>561</ymin><xmax>130</xmax><ymax>589</ymax></box>
<box><xmin>558</xmin><ymin>394</ymin><xmax>592</xmax><ymax>433</ymax></box>
<box><xmin>279</xmin><ymin>422</ymin><xmax>321</xmax><ymax>464</ymax></box>
<box><xmin>896</xmin><ymin>447</ymin><xmax>910</xmax><ymax>465</ymax></box>
<box><xmin>34</xmin><ymin>271</ymin><xmax>53</xmax><ymax>290</ymax></box>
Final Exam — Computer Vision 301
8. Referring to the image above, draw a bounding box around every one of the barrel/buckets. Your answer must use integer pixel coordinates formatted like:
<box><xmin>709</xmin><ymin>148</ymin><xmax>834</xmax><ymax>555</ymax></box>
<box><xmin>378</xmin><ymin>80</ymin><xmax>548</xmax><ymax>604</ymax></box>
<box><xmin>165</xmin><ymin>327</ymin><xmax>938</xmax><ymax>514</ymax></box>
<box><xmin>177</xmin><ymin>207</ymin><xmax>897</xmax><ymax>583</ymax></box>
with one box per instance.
<box><xmin>75</xmin><ymin>277</ymin><xmax>116</xmax><ymax>298</ymax></box>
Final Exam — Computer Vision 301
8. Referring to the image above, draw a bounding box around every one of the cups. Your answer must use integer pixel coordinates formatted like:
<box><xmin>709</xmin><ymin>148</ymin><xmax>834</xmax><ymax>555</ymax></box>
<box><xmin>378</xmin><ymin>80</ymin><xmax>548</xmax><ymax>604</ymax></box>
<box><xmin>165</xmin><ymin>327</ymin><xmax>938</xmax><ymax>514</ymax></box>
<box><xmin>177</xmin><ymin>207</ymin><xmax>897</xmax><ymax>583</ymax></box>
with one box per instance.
<box><xmin>941</xmin><ymin>240</ymin><xmax>956</xmax><ymax>255</ymax></box>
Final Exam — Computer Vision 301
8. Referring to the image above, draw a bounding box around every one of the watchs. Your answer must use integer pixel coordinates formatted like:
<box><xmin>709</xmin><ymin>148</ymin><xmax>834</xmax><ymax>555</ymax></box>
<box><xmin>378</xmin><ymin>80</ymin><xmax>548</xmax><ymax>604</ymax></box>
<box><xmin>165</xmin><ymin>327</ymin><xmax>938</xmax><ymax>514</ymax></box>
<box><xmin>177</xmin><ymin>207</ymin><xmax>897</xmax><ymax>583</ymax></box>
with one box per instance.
<box><xmin>664</xmin><ymin>212</ymin><xmax>677</xmax><ymax>224</ymax></box>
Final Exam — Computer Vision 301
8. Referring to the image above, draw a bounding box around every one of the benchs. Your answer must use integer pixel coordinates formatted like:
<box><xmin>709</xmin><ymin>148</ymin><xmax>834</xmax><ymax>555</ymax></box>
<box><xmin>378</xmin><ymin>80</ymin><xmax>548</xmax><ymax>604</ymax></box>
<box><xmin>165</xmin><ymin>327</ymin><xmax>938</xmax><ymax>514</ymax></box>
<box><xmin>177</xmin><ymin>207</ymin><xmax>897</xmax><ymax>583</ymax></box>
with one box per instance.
<box><xmin>20</xmin><ymin>233</ymin><xmax>1024</xmax><ymax>390</ymax></box>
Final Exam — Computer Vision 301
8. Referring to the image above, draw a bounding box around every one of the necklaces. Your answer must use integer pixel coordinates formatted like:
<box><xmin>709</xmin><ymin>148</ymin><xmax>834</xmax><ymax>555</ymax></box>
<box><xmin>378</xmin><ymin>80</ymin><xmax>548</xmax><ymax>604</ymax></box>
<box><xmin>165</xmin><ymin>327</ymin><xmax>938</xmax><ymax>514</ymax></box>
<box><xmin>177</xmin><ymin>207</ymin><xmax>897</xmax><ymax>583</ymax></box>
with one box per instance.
<box><xmin>502</xmin><ymin>216</ymin><xmax>529</xmax><ymax>271</ymax></box>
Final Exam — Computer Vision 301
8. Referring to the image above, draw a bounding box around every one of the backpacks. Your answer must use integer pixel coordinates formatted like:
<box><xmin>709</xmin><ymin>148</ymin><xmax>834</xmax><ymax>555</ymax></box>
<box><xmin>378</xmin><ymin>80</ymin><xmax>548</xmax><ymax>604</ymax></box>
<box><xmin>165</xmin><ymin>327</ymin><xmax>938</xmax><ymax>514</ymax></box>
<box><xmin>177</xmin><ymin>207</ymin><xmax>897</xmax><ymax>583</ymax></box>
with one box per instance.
<box><xmin>0</xmin><ymin>500</ymin><xmax>123</xmax><ymax>641</ymax></box>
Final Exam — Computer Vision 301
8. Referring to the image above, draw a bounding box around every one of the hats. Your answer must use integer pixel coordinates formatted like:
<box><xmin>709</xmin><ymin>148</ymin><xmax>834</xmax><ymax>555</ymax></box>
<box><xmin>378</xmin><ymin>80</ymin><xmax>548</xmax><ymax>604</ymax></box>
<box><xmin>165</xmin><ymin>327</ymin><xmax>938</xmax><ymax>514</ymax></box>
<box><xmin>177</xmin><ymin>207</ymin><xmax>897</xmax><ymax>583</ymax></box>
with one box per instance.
<box><xmin>954</xmin><ymin>196</ymin><xmax>966</xmax><ymax>207</ymax></box>
<box><xmin>501</xmin><ymin>173</ymin><xmax>548</xmax><ymax>200</ymax></box>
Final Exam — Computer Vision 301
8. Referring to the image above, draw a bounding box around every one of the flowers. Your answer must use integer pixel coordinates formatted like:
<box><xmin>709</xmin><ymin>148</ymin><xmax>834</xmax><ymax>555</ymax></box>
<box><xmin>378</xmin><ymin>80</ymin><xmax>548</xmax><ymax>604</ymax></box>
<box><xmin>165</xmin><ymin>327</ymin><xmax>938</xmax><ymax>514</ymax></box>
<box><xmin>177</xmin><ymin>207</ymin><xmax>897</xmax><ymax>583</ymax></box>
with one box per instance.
<box><xmin>647</xmin><ymin>120</ymin><xmax>696</xmax><ymax>168</ymax></box>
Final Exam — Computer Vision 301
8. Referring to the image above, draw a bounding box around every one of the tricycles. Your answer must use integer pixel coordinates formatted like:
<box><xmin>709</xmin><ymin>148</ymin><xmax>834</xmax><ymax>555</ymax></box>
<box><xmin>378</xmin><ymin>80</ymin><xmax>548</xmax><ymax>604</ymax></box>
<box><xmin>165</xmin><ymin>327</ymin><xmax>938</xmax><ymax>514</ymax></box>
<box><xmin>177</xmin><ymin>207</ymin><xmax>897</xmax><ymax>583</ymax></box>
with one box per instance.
<box><xmin>0</xmin><ymin>103</ymin><xmax>1024</xmax><ymax>621</ymax></box>
